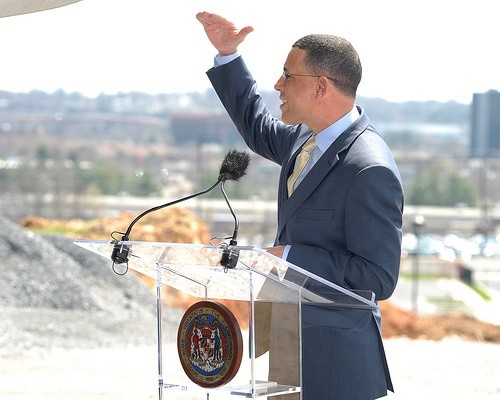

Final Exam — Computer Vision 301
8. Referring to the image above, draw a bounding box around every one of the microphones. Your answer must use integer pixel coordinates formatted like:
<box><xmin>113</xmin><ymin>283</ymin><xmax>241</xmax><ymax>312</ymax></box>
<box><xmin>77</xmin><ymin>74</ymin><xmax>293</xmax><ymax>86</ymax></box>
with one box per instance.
<box><xmin>112</xmin><ymin>149</ymin><xmax>250</xmax><ymax>270</ymax></box>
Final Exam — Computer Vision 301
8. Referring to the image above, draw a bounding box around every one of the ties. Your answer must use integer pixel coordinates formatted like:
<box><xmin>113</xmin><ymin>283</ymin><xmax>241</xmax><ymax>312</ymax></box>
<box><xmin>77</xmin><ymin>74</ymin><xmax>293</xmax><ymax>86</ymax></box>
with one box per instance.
<box><xmin>288</xmin><ymin>135</ymin><xmax>318</xmax><ymax>197</ymax></box>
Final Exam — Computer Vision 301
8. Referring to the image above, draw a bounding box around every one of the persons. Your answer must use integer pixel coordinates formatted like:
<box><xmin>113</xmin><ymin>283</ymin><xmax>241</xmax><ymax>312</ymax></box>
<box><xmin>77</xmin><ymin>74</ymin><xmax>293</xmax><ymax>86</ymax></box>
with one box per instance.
<box><xmin>195</xmin><ymin>10</ymin><xmax>406</xmax><ymax>400</ymax></box>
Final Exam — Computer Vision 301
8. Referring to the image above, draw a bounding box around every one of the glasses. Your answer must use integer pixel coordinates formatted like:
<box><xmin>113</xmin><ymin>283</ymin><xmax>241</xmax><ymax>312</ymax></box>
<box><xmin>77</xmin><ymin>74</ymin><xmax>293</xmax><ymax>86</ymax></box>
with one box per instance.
<box><xmin>281</xmin><ymin>70</ymin><xmax>321</xmax><ymax>82</ymax></box>
